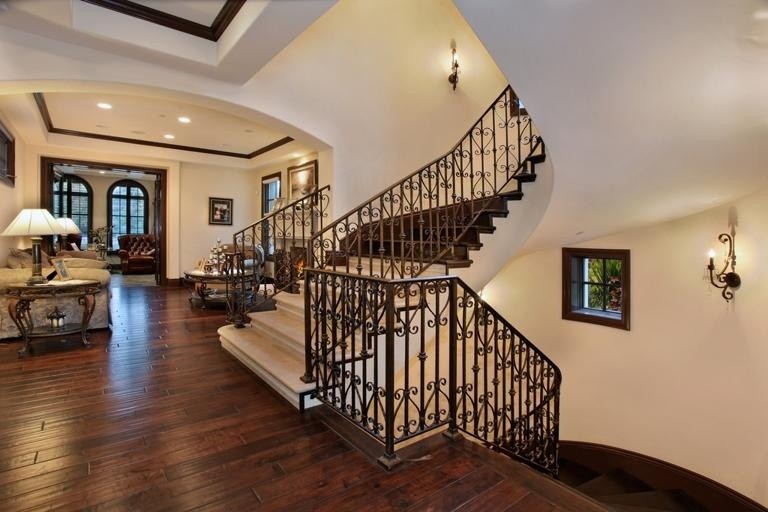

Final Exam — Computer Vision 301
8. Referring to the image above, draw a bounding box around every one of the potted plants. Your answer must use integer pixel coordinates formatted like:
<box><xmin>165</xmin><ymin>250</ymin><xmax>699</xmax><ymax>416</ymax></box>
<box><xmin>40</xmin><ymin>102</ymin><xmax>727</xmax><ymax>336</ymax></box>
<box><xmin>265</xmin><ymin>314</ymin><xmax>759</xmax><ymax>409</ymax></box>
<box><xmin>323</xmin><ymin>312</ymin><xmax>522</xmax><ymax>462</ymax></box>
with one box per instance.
<box><xmin>89</xmin><ymin>224</ymin><xmax>114</xmax><ymax>252</ymax></box>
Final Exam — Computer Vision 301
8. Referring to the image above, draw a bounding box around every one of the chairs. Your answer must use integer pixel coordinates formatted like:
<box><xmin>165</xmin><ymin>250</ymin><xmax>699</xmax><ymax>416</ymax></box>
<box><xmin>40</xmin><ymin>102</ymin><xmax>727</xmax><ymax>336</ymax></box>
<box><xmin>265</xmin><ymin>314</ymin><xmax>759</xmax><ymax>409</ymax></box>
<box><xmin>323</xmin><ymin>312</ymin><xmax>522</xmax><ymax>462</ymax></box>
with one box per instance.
<box><xmin>0</xmin><ymin>234</ymin><xmax>157</xmax><ymax>343</ymax></box>
<box><xmin>216</xmin><ymin>243</ymin><xmax>261</xmax><ymax>295</ymax></box>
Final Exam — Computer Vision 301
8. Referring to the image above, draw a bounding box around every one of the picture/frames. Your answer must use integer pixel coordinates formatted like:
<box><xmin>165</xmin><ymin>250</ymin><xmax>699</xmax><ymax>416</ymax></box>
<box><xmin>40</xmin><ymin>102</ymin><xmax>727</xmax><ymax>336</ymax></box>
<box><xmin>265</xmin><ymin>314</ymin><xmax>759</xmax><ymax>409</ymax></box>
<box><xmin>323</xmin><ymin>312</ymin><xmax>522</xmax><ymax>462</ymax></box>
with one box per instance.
<box><xmin>209</xmin><ymin>158</ymin><xmax>318</xmax><ymax>225</ymax></box>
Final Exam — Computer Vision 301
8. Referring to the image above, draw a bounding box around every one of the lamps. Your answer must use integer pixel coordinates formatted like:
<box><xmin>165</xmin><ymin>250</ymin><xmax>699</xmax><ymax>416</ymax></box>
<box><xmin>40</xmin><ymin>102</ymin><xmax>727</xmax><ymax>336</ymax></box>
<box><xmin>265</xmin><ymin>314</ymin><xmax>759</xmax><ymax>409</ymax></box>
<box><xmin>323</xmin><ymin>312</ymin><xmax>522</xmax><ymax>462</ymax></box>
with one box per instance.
<box><xmin>442</xmin><ymin>36</ymin><xmax>468</xmax><ymax>94</ymax></box>
<box><xmin>1</xmin><ymin>209</ymin><xmax>84</xmax><ymax>284</ymax></box>
<box><xmin>704</xmin><ymin>230</ymin><xmax>740</xmax><ymax>305</ymax></box>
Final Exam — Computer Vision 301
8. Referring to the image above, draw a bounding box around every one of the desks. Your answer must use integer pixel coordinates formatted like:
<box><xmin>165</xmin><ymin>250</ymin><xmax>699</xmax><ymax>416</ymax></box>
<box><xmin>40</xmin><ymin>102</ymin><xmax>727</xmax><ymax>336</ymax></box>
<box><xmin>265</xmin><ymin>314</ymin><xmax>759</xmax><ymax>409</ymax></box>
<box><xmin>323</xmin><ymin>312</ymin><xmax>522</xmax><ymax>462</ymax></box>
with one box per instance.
<box><xmin>183</xmin><ymin>267</ymin><xmax>258</xmax><ymax>311</ymax></box>
<box><xmin>1</xmin><ymin>279</ymin><xmax>102</xmax><ymax>358</ymax></box>
